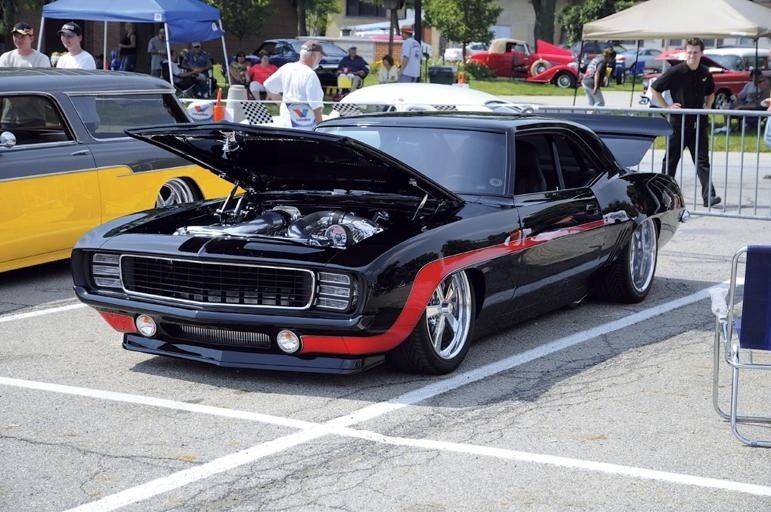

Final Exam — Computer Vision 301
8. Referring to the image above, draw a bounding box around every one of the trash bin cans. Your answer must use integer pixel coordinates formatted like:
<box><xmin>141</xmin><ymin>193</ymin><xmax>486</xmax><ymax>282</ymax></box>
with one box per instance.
<box><xmin>428</xmin><ymin>66</ymin><xmax>456</xmax><ymax>84</ymax></box>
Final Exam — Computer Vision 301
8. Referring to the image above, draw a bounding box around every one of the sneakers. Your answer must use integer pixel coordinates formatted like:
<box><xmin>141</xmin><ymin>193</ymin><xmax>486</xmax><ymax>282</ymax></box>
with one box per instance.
<box><xmin>705</xmin><ymin>196</ymin><xmax>721</xmax><ymax>206</ymax></box>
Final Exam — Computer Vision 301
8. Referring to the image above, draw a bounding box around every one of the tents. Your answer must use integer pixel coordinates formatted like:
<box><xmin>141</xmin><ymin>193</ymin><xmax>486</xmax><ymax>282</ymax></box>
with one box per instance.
<box><xmin>37</xmin><ymin>0</ymin><xmax>231</xmax><ymax>87</ymax></box>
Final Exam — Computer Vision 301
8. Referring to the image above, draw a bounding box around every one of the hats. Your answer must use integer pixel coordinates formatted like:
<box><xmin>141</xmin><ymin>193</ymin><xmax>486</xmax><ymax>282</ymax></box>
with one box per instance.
<box><xmin>56</xmin><ymin>22</ymin><xmax>82</xmax><ymax>36</ymax></box>
<box><xmin>400</xmin><ymin>25</ymin><xmax>413</xmax><ymax>33</ymax></box>
<box><xmin>11</xmin><ymin>22</ymin><xmax>33</xmax><ymax>35</ymax></box>
<box><xmin>301</xmin><ymin>40</ymin><xmax>328</xmax><ymax>56</ymax></box>
<box><xmin>192</xmin><ymin>41</ymin><xmax>200</xmax><ymax>48</ymax></box>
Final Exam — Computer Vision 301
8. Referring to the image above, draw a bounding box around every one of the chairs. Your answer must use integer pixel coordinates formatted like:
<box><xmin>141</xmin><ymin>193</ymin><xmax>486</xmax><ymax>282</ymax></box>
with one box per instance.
<box><xmin>708</xmin><ymin>246</ymin><xmax>771</xmax><ymax>450</ymax></box>
<box><xmin>515</xmin><ymin>140</ymin><xmax>547</xmax><ymax>195</ymax></box>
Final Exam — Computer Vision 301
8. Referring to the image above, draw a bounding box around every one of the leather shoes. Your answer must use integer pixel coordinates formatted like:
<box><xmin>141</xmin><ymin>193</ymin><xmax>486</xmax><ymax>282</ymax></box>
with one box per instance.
<box><xmin>333</xmin><ymin>93</ymin><xmax>343</xmax><ymax>100</ymax></box>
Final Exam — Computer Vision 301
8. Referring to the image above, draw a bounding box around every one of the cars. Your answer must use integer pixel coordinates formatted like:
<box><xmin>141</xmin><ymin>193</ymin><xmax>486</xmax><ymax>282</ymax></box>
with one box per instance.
<box><xmin>327</xmin><ymin>83</ymin><xmax>527</xmax><ymax>116</ymax></box>
<box><xmin>639</xmin><ymin>46</ymin><xmax>771</xmax><ymax>111</ymax></box>
<box><xmin>469</xmin><ymin>39</ymin><xmax>574</xmax><ymax>78</ymax></box>
<box><xmin>235</xmin><ymin>37</ymin><xmax>362</xmax><ymax>86</ymax></box>
<box><xmin>616</xmin><ymin>48</ymin><xmax>664</xmax><ymax>74</ymax></box>
<box><xmin>443</xmin><ymin>41</ymin><xmax>490</xmax><ymax>61</ymax></box>
<box><xmin>569</xmin><ymin>40</ymin><xmax>629</xmax><ymax>74</ymax></box>
<box><xmin>0</xmin><ymin>67</ymin><xmax>247</xmax><ymax>275</ymax></box>
<box><xmin>70</xmin><ymin>114</ymin><xmax>688</xmax><ymax>378</ymax></box>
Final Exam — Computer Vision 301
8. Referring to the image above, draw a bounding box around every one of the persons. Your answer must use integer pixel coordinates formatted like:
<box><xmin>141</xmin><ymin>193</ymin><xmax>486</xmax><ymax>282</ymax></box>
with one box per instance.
<box><xmin>581</xmin><ymin>37</ymin><xmax>771</xmax><ymax>207</ymax></box>
<box><xmin>398</xmin><ymin>25</ymin><xmax>421</xmax><ymax>82</ymax></box>
<box><xmin>56</xmin><ymin>24</ymin><xmax>100</xmax><ymax>121</ymax></box>
<box><xmin>229</xmin><ymin>50</ymin><xmax>279</xmax><ymax>100</ymax></box>
<box><xmin>677</xmin><ymin>120</ymin><xmax>687</xmax><ymax>123</ymax></box>
<box><xmin>264</xmin><ymin>40</ymin><xmax>328</xmax><ymax>130</ymax></box>
<box><xmin>110</xmin><ymin>22</ymin><xmax>212</xmax><ymax>97</ymax></box>
<box><xmin>0</xmin><ymin>21</ymin><xmax>52</xmax><ymax>122</ymax></box>
<box><xmin>332</xmin><ymin>46</ymin><xmax>369</xmax><ymax>101</ymax></box>
<box><xmin>378</xmin><ymin>55</ymin><xmax>398</xmax><ymax>83</ymax></box>
